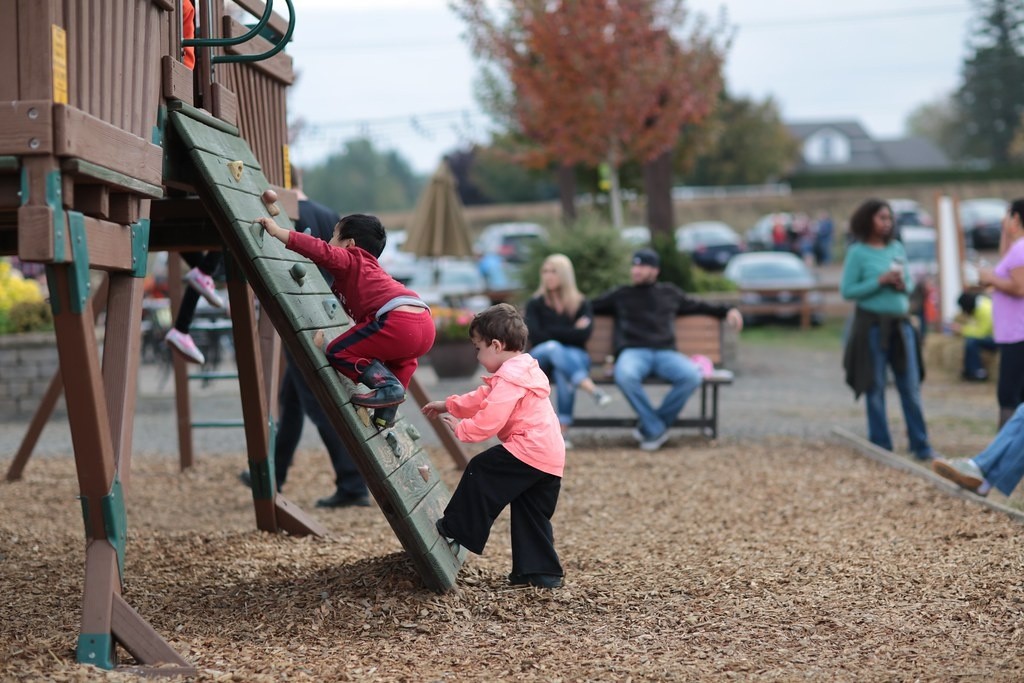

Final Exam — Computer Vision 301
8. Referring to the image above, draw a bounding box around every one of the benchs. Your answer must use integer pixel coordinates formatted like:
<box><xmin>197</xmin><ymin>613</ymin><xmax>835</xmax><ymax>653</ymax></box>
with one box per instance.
<box><xmin>518</xmin><ymin>311</ymin><xmax>737</xmax><ymax>439</ymax></box>
<box><xmin>742</xmin><ymin>288</ymin><xmax>841</xmax><ymax>327</ymax></box>
<box><xmin>441</xmin><ymin>287</ymin><xmax>524</xmax><ymax>305</ymax></box>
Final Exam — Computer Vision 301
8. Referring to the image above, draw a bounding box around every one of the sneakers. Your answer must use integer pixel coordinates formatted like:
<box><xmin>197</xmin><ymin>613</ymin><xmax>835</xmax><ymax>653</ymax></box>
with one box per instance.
<box><xmin>163</xmin><ymin>327</ymin><xmax>206</xmax><ymax>365</ymax></box>
<box><xmin>183</xmin><ymin>267</ymin><xmax>223</xmax><ymax>309</ymax></box>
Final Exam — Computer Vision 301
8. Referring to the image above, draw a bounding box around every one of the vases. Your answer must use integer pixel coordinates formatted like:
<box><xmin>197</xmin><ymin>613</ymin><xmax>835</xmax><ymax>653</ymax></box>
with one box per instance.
<box><xmin>426</xmin><ymin>337</ymin><xmax>480</xmax><ymax>380</ymax></box>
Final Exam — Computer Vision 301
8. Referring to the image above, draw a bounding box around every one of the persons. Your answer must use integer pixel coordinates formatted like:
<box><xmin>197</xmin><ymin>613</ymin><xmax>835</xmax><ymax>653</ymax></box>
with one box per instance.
<box><xmin>978</xmin><ymin>198</ymin><xmax>1024</xmax><ymax>432</ymax></box>
<box><xmin>520</xmin><ymin>252</ymin><xmax>612</xmax><ymax>449</ymax></box>
<box><xmin>841</xmin><ymin>199</ymin><xmax>936</xmax><ymax>461</ymax></box>
<box><xmin>420</xmin><ymin>303</ymin><xmax>566</xmax><ymax>588</ymax></box>
<box><xmin>164</xmin><ymin>251</ymin><xmax>223</xmax><ymax>365</ymax></box>
<box><xmin>773</xmin><ymin>213</ymin><xmax>833</xmax><ymax>268</ymax></box>
<box><xmin>934</xmin><ymin>402</ymin><xmax>1024</xmax><ymax>498</ymax></box>
<box><xmin>182</xmin><ymin>0</ymin><xmax>195</xmax><ymax>70</ymax></box>
<box><xmin>253</xmin><ymin>214</ymin><xmax>436</xmax><ymax>428</ymax></box>
<box><xmin>590</xmin><ymin>248</ymin><xmax>743</xmax><ymax>450</ymax></box>
<box><xmin>950</xmin><ymin>293</ymin><xmax>999</xmax><ymax>382</ymax></box>
<box><xmin>238</xmin><ymin>163</ymin><xmax>372</xmax><ymax>507</ymax></box>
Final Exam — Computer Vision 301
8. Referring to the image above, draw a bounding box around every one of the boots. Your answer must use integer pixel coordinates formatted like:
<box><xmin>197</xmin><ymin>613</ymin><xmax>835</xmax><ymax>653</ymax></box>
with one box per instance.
<box><xmin>368</xmin><ymin>400</ymin><xmax>398</xmax><ymax>428</ymax></box>
<box><xmin>350</xmin><ymin>361</ymin><xmax>409</xmax><ymax>407</ymax></box>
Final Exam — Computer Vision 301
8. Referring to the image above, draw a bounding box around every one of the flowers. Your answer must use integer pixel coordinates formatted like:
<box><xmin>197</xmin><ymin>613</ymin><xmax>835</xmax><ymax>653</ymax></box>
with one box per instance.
<box><xmin>430</xmin><ymin>306</ymin><xmax>481</xmax><ymax>337</ymax></box>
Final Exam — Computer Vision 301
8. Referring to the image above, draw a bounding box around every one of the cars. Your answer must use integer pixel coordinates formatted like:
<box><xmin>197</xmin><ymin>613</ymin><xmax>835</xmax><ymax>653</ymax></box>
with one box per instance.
<box><xmin>371</xmin><ymin>225</ymin><xmax>491</xmax><ymax>317</ymax></box>
<box><xmin>722</xmin><ymin>250</ymin><xmax>826</xmax><ymax>331</ymax></box>
<box><xmin>956</xmin><ymin>196</ymin><xmax>1009</xmax><ymax>251</ymax></box>
<box><xmin>886</xmin><ymin>195</ymin><xmax>981</xmax><ymax>295</ymax></box>
<box><xmin>673</xmin><ymin>221</ymin><xmax>747</xmax><ymax>271</ymax></box>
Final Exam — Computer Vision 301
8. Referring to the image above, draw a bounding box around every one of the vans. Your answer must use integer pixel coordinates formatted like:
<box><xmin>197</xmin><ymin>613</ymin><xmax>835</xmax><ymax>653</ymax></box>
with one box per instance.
<box><xmin>470</xmin><ymin>220</ymin><xmax>551</xmax><ymax>292</ymax></box>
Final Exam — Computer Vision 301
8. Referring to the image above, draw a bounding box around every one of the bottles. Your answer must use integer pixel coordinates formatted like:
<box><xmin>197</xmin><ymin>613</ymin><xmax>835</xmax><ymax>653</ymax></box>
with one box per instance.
<box><xmin>601</xmin><ymin>355</ymin><xmax>614</xmax><ymax>378</ymax></box>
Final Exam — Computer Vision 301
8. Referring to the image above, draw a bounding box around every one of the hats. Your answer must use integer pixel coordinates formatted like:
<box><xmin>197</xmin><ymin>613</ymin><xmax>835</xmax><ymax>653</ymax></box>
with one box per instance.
<box><xmin>631</xmin><ymin>248</ymin><xmax>662</xmax><ymax>269</ymax></box>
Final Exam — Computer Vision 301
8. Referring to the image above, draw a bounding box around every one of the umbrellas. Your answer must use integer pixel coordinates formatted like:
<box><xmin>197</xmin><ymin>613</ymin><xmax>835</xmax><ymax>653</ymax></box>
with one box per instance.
<box><xmin>403</xmin><ymin>160</ymin><xmax>476</xmax><ymax>261</ymax></box>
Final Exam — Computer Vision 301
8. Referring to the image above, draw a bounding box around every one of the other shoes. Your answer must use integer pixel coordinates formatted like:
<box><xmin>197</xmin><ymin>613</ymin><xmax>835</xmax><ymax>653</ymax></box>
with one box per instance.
<box><xmin>434</xmin><ymin>516</ymin><xmax>481</xmax><ymax>553</ymax></box>
<box><xmin>630</xmin><ymin>426</ymin><xmax>647</xmax><ymax>444</ymax></box>
<box><xmin>931</xmin><ymin>455</ymin><xmax>985</xmax><ymax>488</ymax></box>
<box><xmin>636</xmin><ymin>430</ymin><xmax>673</xmax><ymax>452</ymax></box>
<box><xmin>908</xmin><ymin>440</ymin><xmax>931</xmax><ymax>460</ymax></box>
<box><xmin>314</xmin><ymin>492</ymin><xmax>371</xmax><ymax>508</ymax></box>
<box><xmin>588</xmin><ymin>387</ymin><xmax>612</xmax><ymax>411</ymax></box>
<box><xmin>235</xmin><ymin>468</ymin><xmax>283</xmax><ymax>495</ymax></box>
<box><xmin>507</xmin><ymin>572</ymin><xmax>563</xmax><ymax>587</ymax></box>
